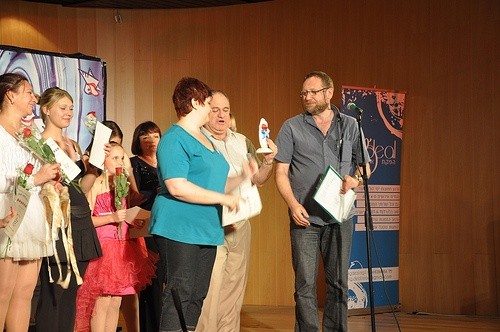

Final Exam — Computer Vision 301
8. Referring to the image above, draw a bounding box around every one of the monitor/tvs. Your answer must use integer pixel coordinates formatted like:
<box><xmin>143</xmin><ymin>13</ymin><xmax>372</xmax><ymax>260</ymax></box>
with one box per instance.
<box><xmin>0</xmin><ymin>43</ymin><xmax>107</xmax><ymax>155</ymax></box>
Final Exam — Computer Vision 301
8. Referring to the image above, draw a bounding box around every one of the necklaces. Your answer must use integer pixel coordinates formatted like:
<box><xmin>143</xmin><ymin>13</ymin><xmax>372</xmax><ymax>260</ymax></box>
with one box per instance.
<box><xmin>10</xmin><ymin>125</ymin><xmax>20</xmax><ymax>136</ymax></box>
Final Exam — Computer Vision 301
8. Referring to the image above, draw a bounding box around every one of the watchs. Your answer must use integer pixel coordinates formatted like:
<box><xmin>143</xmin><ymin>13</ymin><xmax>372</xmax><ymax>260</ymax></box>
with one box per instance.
<box><xmin>263</xmin><ymin>159</ymin><xmax>272</xmax><ymax>165</ymax></box>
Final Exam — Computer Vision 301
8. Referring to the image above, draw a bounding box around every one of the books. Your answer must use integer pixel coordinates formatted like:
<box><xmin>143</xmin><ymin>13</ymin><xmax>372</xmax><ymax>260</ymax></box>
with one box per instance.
<box><xmin>313</xmin><ymin>165</ymin><xmax>357</xmax><ymax>224</ymax></box>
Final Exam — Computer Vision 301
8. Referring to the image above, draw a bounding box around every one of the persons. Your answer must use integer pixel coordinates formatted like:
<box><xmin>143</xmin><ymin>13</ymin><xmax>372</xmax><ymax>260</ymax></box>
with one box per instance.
<box><xmin>73</xmin><ymin>141</ymin><xmax>160</xmax><ymax>332</ymax></box>
<box><xmin>0</xmin><ymin>73</ymin><xmax>61</xmax><ymax>332</ymax></box>
<box><xmin>274</xmin><ymin>70</ymin><xmax>371</xmax><ymax>332</ymax></box>
<box><xmin>128</xmin><ymin>121</ymin><xmax>170</xmax><ymax>332</ymax></box>
<box><xmin>71</xmin><ymin>120</ymin><xmax>142</xmax><ymax>332</ymax></box>
<box><xmin>193</xmin><ymin>89</ymin><xmax>277</xmax><ymax>332</ymax></box>
<box><xmin>34</xmin><ymin>86</ymin><xmax>112</xmax><ymax>332</ymax></box>
<box><xmin>0</xmin><ymin>206</ymin><xmax>15</xmax><ymax>228</ymax></box>
<box><xmin>146</xmin><ymin>77</ymin><xmax>258</xmax><ymax>332</ymax></box>
<box><xmin>228</xmin><ymin>113</ymin><xmax>237</xmax><ymax>132</ymax></box>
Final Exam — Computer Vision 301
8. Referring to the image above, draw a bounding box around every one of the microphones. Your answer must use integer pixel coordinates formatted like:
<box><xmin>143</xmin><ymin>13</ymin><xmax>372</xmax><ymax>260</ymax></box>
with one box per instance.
<box><xmin>347</xmin><ymin>102</ymin><xmax>363</xmax><ymax>115</ymax></box>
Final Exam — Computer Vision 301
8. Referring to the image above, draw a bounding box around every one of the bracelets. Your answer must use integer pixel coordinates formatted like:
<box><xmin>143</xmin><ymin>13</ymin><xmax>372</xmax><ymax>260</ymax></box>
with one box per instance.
<box><xmin>354</xmin><ymin>174</ymin><xmax>360</xmax><ymax>182</ymax></box>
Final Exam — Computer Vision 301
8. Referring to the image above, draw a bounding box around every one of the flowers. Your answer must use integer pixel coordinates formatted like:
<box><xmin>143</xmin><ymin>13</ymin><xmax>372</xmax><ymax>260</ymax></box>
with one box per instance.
<box><xmin>15</xmin><ymin>126</ymin><xmax>83</xmax><ymax>195</ymax></box>
<box><xmin>14</xmin><ymin>235</ymin><xmax>18</xmax><ymax>238</ymax></box>
<box><xmin>0</xmin><ymin>163</ymin><xmax>34</xmax><ymax>262</ymax></box>
<box><xmin>112</xmin><ymin>167</ymin><xmax>130</xmax><ymax>244</ymax></box>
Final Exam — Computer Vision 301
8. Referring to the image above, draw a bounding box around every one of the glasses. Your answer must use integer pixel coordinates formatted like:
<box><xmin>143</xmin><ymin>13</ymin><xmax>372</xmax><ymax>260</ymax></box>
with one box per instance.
<box><xmin>300</xmin><ymin>87</ymin><xmax>329</xmax><ymax>96</ymax></box>
